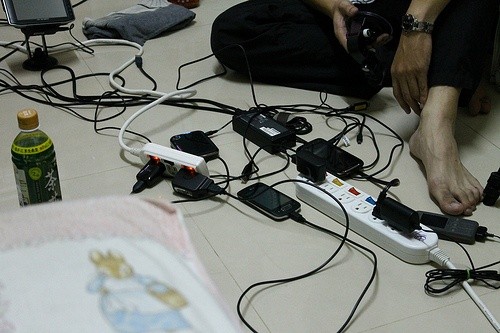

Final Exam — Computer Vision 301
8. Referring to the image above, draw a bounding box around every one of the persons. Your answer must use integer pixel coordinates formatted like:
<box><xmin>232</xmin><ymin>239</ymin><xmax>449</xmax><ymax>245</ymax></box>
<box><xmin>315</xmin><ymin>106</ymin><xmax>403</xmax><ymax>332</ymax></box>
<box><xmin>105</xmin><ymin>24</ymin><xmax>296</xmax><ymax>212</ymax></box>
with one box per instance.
<box><xmin>209</xmin><ymin>0</ymin><xmax>500</xmax><ymax>216</ymax></box>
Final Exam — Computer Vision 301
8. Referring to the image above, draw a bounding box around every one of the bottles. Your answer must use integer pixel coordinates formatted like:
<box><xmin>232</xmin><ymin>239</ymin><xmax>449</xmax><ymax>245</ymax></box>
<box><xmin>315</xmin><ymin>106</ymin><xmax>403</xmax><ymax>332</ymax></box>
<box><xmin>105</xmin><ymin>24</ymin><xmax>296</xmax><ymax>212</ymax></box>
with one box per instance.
<box><xmin>11</xmin><ymin>110</ymin><xmax>62</xmax><ymax>207</ymax></box>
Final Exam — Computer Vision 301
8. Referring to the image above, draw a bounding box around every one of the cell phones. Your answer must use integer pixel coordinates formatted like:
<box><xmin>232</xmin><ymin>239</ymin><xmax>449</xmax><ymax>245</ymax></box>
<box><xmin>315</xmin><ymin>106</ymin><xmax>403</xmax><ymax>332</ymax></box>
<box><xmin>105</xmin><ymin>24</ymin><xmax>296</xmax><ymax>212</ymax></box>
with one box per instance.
<box><xmin>411</xmin><ymin>210</ymin><xmax>479</xmax><ymax>245</ymax></box>
<box><xmin>236</xmin><ymin>182</ymin><xmax>302</xmax><ymax>221</ymax></box>
<box><xmin>170</xmin><ymin>130</ymin><xmax>219</xmax><ymax>161</ymax></box>
<box><xmin>296</xmin><ymin>137</ymin><xmax>363</xmax><ymax>180</ymax></box>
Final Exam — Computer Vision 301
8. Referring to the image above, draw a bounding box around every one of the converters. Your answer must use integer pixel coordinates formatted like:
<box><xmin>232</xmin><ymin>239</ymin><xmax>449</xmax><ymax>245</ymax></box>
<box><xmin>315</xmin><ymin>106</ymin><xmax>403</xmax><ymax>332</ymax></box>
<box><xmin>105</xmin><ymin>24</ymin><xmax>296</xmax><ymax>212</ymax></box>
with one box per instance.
<box><xmin>232</xmin><ymin>110</ymin><xmax>297</xmax><ymax>155</ymax></box>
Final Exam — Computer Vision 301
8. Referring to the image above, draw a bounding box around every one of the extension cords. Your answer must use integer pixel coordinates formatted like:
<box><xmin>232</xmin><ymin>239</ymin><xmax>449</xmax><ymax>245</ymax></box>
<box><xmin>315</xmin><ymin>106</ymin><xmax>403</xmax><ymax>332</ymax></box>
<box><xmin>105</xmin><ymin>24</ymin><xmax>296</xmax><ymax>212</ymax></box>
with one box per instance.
<box><xmin>139</xmin><ymin>143</ymin><xmax>209</xmax><ymax>181</ymax></box>
<box><xmin>294</xmin><ymin>169</ymin><xmax>439</xmax><ymax>264</ymax></box>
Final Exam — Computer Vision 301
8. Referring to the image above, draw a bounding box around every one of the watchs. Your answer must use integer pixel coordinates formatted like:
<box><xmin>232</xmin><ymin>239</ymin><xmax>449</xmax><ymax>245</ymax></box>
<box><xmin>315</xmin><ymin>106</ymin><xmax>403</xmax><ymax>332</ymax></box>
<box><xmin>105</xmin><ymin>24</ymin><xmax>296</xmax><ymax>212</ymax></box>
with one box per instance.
<box><xmin>400</xmin><ymin>11</ymin><xmax>434</xmax><ymax>35</ymax></box>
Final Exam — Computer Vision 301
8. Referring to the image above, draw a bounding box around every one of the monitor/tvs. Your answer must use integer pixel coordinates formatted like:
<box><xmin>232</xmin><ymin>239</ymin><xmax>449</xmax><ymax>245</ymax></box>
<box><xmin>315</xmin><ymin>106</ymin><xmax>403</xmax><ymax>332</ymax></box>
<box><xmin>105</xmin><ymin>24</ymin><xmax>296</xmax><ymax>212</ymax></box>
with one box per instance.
<box><xmin>3</xmin><ymin>0</ymin><xmax>75</xmax><ymax>26</ymax></box>
